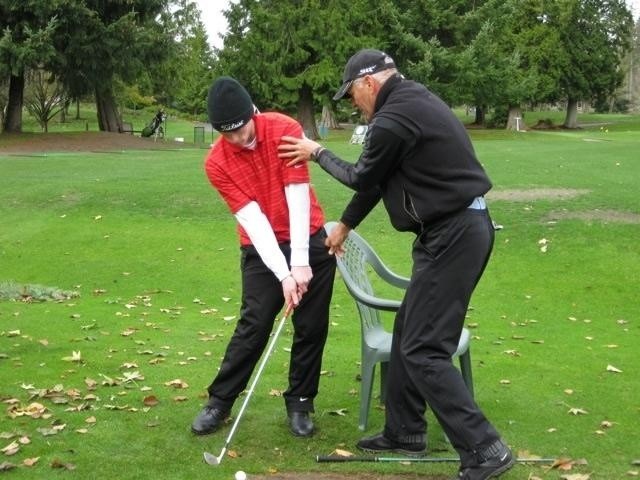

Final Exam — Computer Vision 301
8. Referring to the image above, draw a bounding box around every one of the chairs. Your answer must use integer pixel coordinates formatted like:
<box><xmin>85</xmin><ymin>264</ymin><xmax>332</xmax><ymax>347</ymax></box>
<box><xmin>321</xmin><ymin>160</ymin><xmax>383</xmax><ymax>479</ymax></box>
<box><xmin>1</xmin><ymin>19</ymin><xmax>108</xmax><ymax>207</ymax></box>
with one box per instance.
<box><xmin>324</xmin><ymin>220</ymin><xmax>474</xmax><ymax>433</ymax></box>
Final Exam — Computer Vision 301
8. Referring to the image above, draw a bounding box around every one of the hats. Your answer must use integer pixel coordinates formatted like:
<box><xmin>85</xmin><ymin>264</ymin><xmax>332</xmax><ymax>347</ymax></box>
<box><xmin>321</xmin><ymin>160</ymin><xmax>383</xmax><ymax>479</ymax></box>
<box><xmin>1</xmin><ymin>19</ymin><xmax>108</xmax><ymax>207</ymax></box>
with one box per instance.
<box><xmin>207</xmin><ymin>77</ymin><xmax>254</xmax><ymax>132</ymax></box>
<box><xmin>333</xmin><ymin>47</ymin><xmax>396</xmax><ymax>102</ymax></box>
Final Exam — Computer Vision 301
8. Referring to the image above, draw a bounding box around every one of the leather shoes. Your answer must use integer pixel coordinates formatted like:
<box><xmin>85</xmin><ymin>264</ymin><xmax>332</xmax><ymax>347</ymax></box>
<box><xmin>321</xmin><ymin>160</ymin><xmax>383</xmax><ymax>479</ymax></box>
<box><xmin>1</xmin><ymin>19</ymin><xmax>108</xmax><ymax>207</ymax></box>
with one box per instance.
<box><xmin>191</xmin><ymin>406</ymin><xmax>232</xmax><ymax>435</ymax></box>
<box><xmin>286</xmin><ymin>411</ymin><xmax>313</xmax><ymax>437</ymax></box>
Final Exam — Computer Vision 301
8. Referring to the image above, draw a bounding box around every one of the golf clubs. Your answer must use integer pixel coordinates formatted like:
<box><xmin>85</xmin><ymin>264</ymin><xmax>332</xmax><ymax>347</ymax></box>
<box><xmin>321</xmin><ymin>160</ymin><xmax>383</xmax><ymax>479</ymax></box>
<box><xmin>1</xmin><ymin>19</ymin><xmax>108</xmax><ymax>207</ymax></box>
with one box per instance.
<box><xmin>156</xmin><ymin>108</ymin><xmax>166</xmax><ymax>122</ymax></box>
<box><xmin>316</xmin><ymin>455</ymin><xmax>572</xmax><ymax>472</ymax></box>
<box><xmin>203</xmin><ymin>301</ymin><xmax>293</xmax><ymax>467</ymax></box>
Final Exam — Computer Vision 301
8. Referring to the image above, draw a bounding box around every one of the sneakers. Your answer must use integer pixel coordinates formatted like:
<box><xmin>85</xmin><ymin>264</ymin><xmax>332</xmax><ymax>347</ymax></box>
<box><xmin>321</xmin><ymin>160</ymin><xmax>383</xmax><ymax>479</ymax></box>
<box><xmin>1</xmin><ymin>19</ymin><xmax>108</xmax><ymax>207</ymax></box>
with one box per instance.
<box><xmin>356</xmin><ymin>434</ymin><xmax>427</xmax><ymax>458</ymax></box>
<box><xmin>455</xmin><ymin>446</ymin><xmax>514</xmax><ymax>480</ymax></box>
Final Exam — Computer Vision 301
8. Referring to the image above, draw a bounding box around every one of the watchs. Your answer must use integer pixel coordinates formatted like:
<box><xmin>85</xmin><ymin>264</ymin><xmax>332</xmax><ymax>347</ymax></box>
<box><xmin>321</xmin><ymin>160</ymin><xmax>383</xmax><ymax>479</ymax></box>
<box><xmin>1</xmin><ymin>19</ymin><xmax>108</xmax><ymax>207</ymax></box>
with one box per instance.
<box><xmin>310</xmin><ymin>147</ymin><xmax>323</xmax><ymax>162</ymax></box>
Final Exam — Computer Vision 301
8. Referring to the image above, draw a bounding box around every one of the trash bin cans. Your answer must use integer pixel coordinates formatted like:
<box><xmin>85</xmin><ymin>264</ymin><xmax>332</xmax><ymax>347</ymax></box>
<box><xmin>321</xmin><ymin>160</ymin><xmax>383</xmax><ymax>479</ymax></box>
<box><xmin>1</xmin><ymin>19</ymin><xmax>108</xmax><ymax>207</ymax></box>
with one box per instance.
<box><xmin>318</xmin><ymin>122</ymin><xmax>328</xmax><ymax>139</ymax></box>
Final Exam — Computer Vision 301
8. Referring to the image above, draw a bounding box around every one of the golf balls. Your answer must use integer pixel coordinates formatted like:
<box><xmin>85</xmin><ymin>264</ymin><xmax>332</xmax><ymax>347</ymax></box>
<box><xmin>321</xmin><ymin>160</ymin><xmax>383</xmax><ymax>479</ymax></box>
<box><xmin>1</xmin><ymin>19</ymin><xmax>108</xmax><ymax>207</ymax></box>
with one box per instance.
<box><xmin>236</xmin><ymin>471</ymin><xmax>246</xmax><ymax>480</ymax></box>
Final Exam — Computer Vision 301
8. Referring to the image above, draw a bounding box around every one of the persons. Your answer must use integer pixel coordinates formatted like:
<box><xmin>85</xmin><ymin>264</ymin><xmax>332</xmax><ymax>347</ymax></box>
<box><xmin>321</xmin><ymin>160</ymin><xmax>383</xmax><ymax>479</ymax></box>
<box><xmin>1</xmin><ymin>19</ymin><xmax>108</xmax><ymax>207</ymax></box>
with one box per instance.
<box><xmin>191</xmin><ymin>77</ymin><xmax>337</xmax><ymax>437</ymax></box>
<box><xmin>278</xmin><ymin>49</ymin><xmax>515</xmax><ymax>480</ymax></box>
